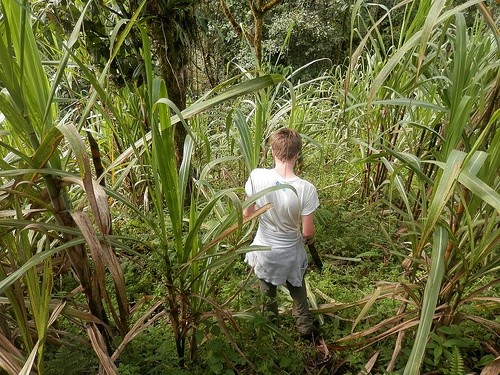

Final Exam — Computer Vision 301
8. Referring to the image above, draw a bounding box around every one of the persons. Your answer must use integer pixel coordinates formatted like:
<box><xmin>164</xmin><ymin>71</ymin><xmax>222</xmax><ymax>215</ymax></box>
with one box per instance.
<box><xmin>243</xmin><ymin>127</ymin><xmax>323</xmax><ymax>342</ymax></box>
